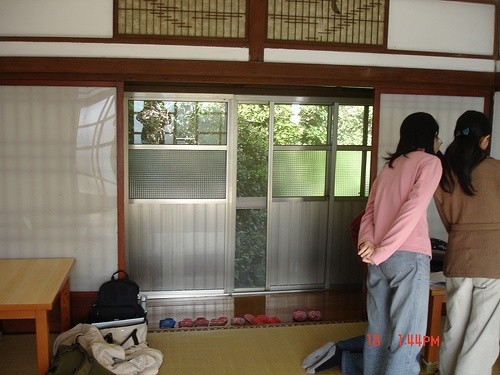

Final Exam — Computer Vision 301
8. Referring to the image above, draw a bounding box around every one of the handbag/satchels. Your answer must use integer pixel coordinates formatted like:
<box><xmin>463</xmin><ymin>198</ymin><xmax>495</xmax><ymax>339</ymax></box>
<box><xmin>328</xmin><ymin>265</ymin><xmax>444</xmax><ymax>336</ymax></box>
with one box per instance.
<box><xmin>45</xmin><ymin>344</ymin><xmax>96</xmax><ymax>374</ymax></box>
<box><xmin>90</xmin><ymin>317</ymin><xmax>149</xmax><ymax>350</ymax></box>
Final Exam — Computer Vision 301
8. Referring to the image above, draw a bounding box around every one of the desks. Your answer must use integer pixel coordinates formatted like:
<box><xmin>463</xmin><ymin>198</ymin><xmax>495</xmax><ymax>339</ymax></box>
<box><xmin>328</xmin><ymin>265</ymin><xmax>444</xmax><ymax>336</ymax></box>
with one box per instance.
<box><xmin>420</xmin><ymin>271</ymin><xmax>447</xmax><ymax>375</ymax></box>
<box><xmin>0</xmin><ymin>258</ymin><xmax>76</xmax><ymax>375</ymax></box>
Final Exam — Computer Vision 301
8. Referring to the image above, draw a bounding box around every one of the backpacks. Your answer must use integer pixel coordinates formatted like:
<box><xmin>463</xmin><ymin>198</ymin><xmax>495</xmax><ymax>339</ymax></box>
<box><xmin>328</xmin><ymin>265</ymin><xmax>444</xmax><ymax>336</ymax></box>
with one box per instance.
<box><xmin>88</xmin><ymin>269</ymin><xmax>149</xmax><ymax>325</ymax></box>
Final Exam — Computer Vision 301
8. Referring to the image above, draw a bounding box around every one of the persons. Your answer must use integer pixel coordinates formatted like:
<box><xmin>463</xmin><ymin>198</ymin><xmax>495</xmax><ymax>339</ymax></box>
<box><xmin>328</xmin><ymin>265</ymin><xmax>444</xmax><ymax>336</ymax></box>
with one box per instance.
<box><xmin>434</xmin><ymin>110</ymin><xmax>500</xmax><ymax>375</ymax></box>
<box><xmin>303</xmin><ymin>235</ymin><xmax>448</xmax><ymax>375</ymax></box>
<box><xmin>357</xmin><ymin>112</ymin><xmax>443</xmax><ymax>375</ymax></box>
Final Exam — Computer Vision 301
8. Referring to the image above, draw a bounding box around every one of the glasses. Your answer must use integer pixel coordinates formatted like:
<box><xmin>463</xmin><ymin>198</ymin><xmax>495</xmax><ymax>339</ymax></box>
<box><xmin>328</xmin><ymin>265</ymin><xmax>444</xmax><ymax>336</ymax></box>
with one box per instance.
<box><xmin>437</xmin><ymin>136</ymin><xmax>443</xmax><ymax>146</ymax></box>
